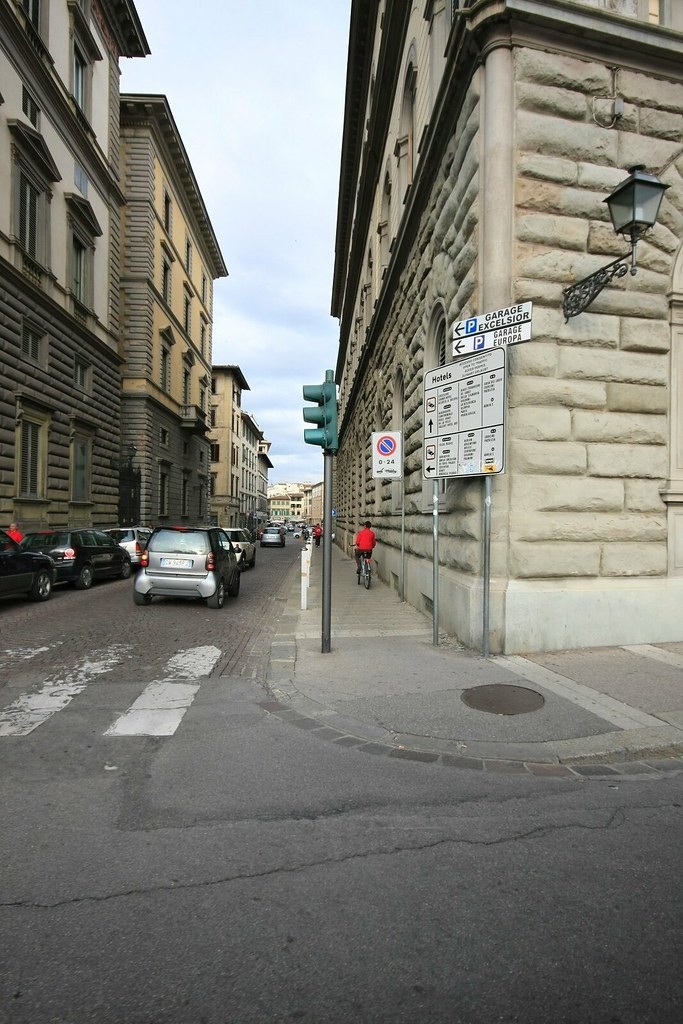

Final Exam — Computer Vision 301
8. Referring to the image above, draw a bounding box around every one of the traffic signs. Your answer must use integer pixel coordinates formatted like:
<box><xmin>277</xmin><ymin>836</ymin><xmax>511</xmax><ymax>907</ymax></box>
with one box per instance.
<box><xmin>452</xmin><ymin>301</ymin><xmax>532</xmax><ymax>338</ymax></box>
<box><xmin>452</xmin><ymin>322</ymin><xmax>531</xmax><ymax>355</ymax></box>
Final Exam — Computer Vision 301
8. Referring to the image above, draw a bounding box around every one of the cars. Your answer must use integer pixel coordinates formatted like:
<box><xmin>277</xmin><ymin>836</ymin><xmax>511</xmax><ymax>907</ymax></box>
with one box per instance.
<box><xmin>98</xmin><ymin>526</ymin><xmax>154</xmax><ymax>567</ymax></box>
<box><xmin>133</xmin><ymin>522</ymin><xmax>242</xmax><ymax>609</ymax></box>
<box><xmin>214</xmin><ymin>527</ymin><xmax>256</xmax><ymax>572</ymax></box>
<box><xmin>293</xmin><ymin>526</ymin><xmax>314</xmax><ymax>539</ymax></box>
<box><xmin>265</xmin><ymin>519</ymin><xmax>308</xmax><ymax>531</ymax></box>
<box><xmin>0</xmin><ymin>529</ymin><xmax>59</xmax><ymax>602</ymax></box>
<box><xmin>16</xmin><ymin>526</ymin><xmax>134</xmax><ymax>590</ymax></box>
<box><xmin>260</xmin><ymin>526</ymin><xmax>287</xmax><ymax>547</ymax></box>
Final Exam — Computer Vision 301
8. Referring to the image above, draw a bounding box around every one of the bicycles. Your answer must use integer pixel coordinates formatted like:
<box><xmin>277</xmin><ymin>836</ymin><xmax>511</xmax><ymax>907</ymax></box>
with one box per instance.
<box><xmin>350</xmin><ymin>543</ymin><xmax>373</xmax><ymax>589</ymax></box>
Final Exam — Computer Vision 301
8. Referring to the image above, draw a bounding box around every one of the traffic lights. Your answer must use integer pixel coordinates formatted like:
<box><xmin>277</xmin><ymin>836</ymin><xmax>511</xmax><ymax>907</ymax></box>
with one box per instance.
<box><xmin>302</xmin><ymin>379</ymin><xmax>339</xmax><ymax>454</ymax></box>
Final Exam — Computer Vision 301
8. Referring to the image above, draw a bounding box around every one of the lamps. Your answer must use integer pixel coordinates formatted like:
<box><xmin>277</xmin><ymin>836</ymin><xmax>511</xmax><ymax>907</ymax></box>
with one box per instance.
<box><xmin>561</xmin><ymin>164</ymin><xmax>671</xmax><ymax>325</ymax></box>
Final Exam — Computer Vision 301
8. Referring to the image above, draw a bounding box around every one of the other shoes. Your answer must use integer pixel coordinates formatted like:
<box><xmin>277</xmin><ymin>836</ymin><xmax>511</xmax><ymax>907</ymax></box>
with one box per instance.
<box><xmin>356</xmin><ymin>571</ymin><xmax>360</xmax><ymax>576</ymax></box>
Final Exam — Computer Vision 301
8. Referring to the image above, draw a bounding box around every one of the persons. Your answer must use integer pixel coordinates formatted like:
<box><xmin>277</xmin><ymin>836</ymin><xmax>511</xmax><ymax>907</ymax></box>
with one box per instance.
<box><xmin>314</xmin><ymin>523</ymin><xmax>322</xmax><ymax>548</ymax></box>
<box><xmin>6</xmin><ymin>522</ymin><xmax>24</xmax><ymax>543</ymax></box>
<box><xmin>355</xmin><ymin>520</ymin><xmax>377</xmax><ymax>574</ymax></box>
